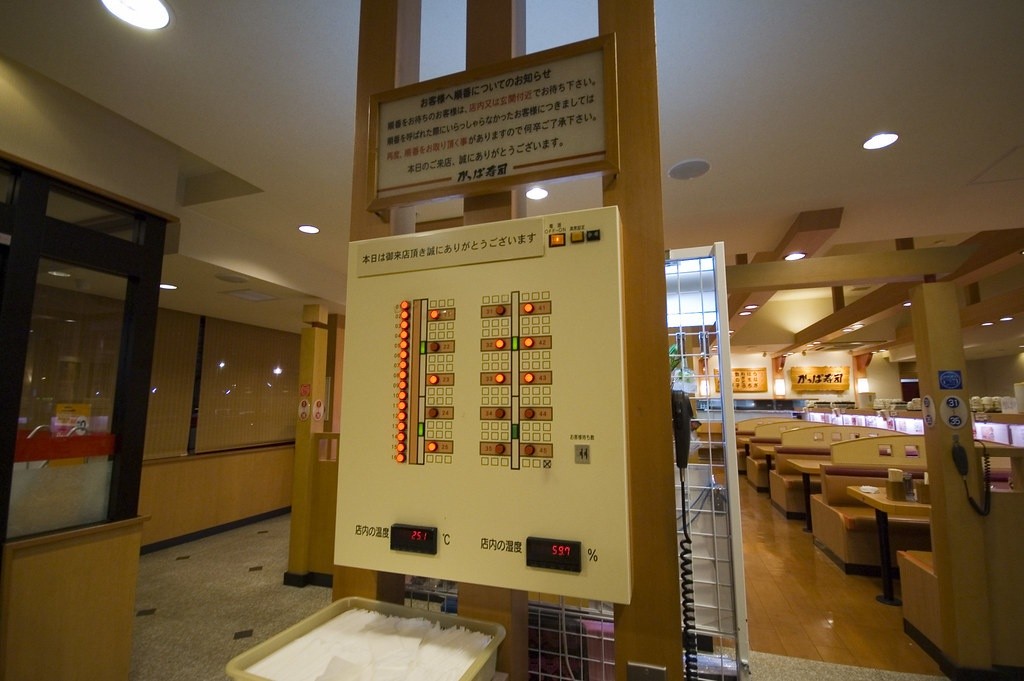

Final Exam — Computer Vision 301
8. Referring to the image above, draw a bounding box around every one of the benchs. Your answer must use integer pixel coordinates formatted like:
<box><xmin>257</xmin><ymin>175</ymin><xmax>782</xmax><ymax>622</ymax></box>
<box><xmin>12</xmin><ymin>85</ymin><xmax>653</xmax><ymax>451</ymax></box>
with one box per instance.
<box><xmin>735</xmin><ymin>417</ymin><xmax>1024</xmax><ymax>681</ymax></box>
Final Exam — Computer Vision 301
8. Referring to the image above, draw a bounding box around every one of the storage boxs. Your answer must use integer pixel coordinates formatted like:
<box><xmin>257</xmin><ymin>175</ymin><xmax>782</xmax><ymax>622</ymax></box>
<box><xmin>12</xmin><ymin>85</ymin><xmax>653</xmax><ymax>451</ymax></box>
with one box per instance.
<box><xmin>225</xmin><ymin>596</ymin><xmax>507</xmax><ymax>680</ymax></box>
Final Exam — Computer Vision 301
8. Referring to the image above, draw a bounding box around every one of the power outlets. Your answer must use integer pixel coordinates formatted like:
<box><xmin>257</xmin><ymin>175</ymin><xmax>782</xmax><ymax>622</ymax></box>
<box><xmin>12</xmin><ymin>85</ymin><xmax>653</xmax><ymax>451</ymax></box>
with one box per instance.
<box><xmin>626</xmin><ymin>661</ymin><xmax>667</xmax><ymax>681</ymax></box>
